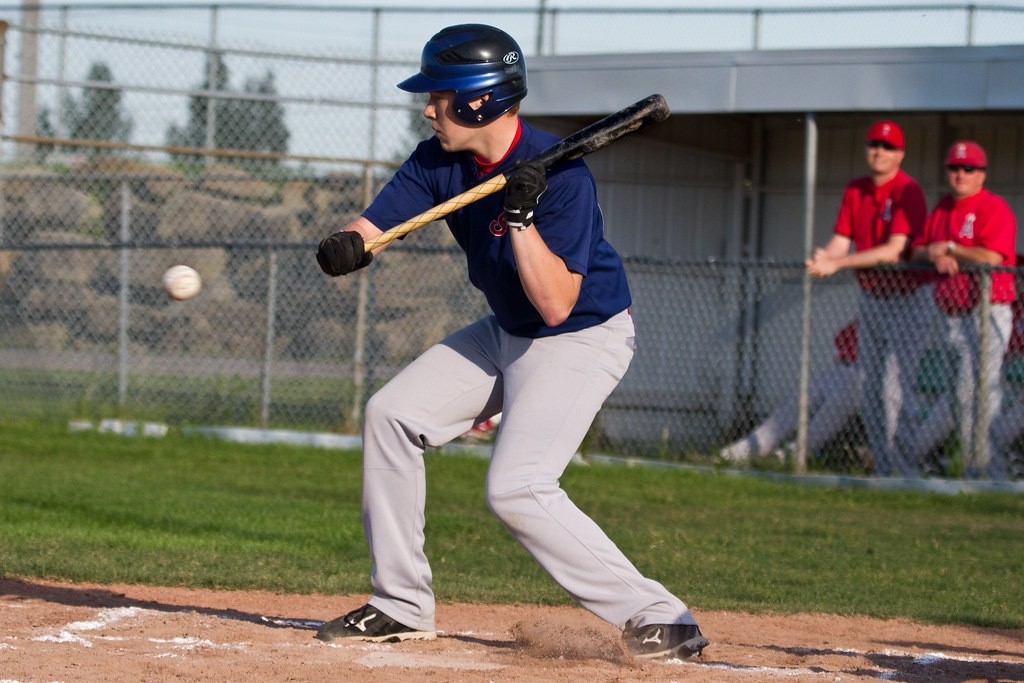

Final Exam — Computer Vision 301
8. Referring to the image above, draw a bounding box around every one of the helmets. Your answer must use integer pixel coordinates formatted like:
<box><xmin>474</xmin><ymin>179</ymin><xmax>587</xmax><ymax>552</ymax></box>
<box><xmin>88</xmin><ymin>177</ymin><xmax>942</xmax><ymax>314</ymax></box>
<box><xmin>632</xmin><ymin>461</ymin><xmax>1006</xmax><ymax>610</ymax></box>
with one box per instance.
<box><xmin>397</xmin><ymin>24</ymin><xmax>528</xmax><ymax>124</ymax></box>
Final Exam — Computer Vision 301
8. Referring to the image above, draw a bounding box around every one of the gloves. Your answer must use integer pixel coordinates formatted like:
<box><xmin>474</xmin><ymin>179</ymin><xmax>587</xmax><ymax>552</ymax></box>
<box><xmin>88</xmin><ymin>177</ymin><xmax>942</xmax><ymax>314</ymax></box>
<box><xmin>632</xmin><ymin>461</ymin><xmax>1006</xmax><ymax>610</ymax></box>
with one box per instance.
<box><xmin>316</xmin><ymin>230</ymin><xmax>373</xmax><ymax>277</ymax></box>
<box><xmin>502</xmin><ymin>158</ymin><xmax>548</xmax><ymax>231</ymax></box>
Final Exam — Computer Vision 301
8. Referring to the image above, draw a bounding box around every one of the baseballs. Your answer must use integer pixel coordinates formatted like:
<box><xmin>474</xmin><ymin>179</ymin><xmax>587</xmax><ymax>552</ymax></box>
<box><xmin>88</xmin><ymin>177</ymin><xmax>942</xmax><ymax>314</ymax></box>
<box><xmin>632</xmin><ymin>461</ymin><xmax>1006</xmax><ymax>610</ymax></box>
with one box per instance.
<box><xmin>162</xmin><ymin>264</ymin><xmax>203</xmax><ymax>301</ymax></box>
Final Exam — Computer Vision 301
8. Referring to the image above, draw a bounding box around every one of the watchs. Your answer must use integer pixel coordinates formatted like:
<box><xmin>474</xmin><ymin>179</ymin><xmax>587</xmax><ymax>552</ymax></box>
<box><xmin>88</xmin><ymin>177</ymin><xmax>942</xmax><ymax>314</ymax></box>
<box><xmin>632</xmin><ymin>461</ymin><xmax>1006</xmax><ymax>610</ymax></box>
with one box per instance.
<box><xmin>946</xmin><ymin>240</ymin><xmax>956</xmax><ymax>255</ymax></box>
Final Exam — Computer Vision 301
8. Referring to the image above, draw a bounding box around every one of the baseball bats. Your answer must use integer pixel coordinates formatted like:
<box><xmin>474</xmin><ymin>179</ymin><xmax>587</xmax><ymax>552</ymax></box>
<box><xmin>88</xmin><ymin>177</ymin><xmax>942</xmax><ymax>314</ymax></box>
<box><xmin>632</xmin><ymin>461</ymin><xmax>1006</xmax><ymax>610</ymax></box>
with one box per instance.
<box><xmin>315</xmin><ymin>92</ymin><xmax>672</xmax><ymax>266</ymax></box>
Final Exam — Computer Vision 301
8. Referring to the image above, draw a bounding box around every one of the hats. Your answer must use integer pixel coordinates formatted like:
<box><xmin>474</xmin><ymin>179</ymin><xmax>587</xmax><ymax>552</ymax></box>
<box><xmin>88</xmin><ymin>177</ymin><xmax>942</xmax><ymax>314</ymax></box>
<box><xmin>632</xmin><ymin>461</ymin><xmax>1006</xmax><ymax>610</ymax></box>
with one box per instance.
<box><xmin>945</xmin><ymin>141</ymin><xmax>988</xmax><ymax>168</ymax></box>
<box><xmin>864</xmin><ymin>120</ymin><xmax>906</xmax><ymax>149</ymax></box>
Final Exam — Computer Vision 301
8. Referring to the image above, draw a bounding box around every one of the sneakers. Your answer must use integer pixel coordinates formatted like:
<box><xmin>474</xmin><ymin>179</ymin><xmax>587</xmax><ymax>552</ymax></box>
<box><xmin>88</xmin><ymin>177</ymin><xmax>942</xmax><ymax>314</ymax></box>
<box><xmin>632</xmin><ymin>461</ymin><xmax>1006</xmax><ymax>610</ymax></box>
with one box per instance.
<box><xmin>615</xmin><ymin>619</ymin><xmax>710</xmax><ymax>658</ymax></box>
<box><xmin>316</xmin><ymin>604</ymin><xmax>436</xmax><ymax>641</ymax></box>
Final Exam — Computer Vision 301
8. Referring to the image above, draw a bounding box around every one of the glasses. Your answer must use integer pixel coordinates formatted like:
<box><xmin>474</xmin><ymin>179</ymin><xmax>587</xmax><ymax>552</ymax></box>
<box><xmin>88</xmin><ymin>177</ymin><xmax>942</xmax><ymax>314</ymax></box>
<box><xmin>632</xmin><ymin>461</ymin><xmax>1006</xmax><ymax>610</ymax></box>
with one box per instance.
<box><xmin>948</xmin><ymin>166</ymin><xmax>974</xmax><ymax>173</ymax></box>
<box><xmin>866</xmin><ymin>142</ymin><xmax>894</xmax><ymax>150</ymax></box>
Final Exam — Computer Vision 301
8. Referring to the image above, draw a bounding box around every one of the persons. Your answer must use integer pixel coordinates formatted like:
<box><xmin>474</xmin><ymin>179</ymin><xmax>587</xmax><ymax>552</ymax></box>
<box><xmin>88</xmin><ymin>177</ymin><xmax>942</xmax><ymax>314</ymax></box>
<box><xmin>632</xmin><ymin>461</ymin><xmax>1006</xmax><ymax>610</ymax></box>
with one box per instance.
<box><xmin>804</xmin><ymin>119</ymin><xmax>937</xmax><ymax>478</ymax></box>
<box><xmin>910</xmin><ymin>141</ymin><xmax>1018</xmax><ymax>479</ymax></box>
<box><xmin>713</xmin><ymin>316</ymin><xmax>859</xmax><ymax>474</ymax></box>
<box><xmin>314</xmin><ymin>23</ymin><xmax>710</xmax><ymax>663</ymax></box>
